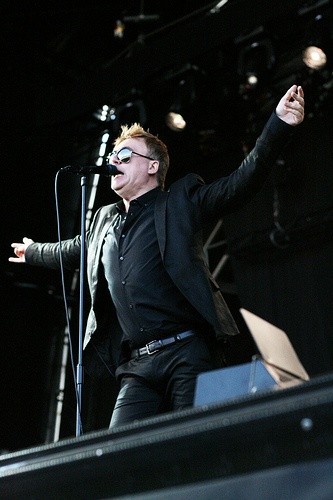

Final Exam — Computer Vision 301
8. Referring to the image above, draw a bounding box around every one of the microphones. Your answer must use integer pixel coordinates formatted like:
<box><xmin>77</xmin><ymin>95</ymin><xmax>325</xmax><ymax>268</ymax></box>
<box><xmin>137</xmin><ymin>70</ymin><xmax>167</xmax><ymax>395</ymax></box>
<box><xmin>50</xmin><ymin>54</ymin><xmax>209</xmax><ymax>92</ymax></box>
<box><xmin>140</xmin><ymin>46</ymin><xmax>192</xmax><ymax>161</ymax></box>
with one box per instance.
<box><xmin>64</xmin><ymin>165</ymin><xmax>117</xmax><ymax>176</ymax></box>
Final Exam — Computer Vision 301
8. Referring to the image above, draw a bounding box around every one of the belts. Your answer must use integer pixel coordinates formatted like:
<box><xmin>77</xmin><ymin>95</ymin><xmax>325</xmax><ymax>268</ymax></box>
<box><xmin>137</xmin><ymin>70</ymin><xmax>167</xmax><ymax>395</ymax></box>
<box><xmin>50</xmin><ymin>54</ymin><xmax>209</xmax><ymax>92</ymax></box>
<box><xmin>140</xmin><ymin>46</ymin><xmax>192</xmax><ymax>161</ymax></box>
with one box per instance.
<box><xmin>128</xmin><ymin>331</ymin><xmax>195</xmax><ymax>357</ymax></box>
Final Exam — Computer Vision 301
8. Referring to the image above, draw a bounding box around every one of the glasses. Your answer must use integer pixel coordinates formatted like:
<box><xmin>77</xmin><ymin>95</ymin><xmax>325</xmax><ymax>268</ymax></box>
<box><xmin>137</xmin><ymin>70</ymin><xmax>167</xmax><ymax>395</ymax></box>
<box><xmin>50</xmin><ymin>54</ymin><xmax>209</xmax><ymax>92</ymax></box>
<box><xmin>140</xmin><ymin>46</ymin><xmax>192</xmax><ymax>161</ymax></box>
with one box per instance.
<box><xmin>106</xmin><ymin>147</ymin><xmax>155</xmax><ymax>165</ymax></box>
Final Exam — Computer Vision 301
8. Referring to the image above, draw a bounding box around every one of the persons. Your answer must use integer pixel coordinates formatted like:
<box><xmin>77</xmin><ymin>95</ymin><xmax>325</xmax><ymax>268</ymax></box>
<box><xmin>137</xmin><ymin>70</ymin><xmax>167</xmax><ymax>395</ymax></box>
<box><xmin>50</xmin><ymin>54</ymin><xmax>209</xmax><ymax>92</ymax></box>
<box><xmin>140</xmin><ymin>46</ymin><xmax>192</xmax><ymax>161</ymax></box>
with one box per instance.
<box><xmin>8</xmin><ymin>84</ymin><xmax>306</xmax><ymax>430</ymax></box>
